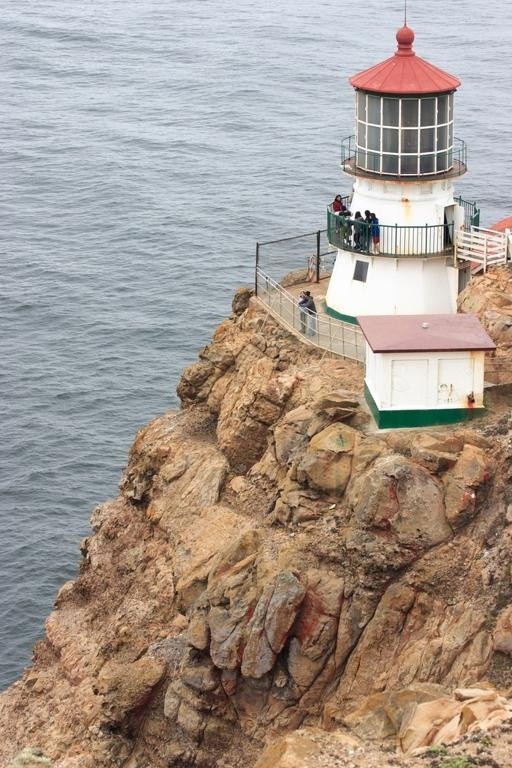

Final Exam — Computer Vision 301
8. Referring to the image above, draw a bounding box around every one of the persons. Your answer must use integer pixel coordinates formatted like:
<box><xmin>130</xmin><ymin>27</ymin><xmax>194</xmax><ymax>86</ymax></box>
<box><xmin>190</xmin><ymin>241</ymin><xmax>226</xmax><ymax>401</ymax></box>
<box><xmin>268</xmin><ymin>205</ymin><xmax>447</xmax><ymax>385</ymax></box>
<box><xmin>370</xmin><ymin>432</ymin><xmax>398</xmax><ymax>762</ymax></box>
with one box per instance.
<box><xmin>332</xmin><ymin>194</ymin><xmax>380</xmax><ymax>255</ymax></box>
<box><xmin>298</xmin><ymin>290</ymin><xmax>306</xmax><ymax>334</ymax></box>
<box><xmin>299</xmin><ymin>291</ymin><xmax>317</xmax><ymax>336</ymax></box>
